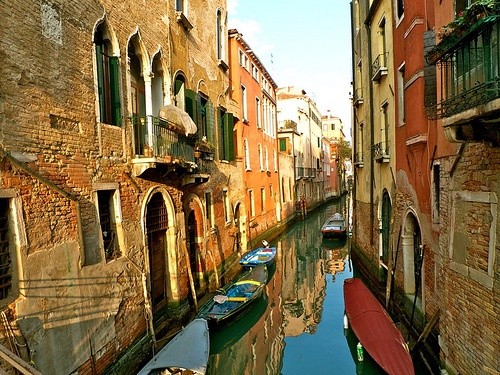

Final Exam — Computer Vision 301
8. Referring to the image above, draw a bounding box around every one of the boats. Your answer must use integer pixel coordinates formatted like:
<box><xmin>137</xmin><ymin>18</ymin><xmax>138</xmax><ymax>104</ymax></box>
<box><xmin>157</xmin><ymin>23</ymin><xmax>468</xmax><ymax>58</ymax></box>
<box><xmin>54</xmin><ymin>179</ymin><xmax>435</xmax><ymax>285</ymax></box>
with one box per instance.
<box><xmin>343</xmin><ymin>278</ymin><xmax>414</xmax><ymax>375</ymax></box>
<box><xmin>136</xmin><ymin>318</ymin><xmax>210</xmax><ymax>375</ymax></box>
<box><xmin>321</xmin><ymin>213</ymin><xmax>346</xmax><ymax>237</ymax></box>
<box><xmin>239</xmin><ymin>247</ymin><xmax>276</xmax><ymax>267</ymax></box>
<box><xmin>195</xmin><ymin>264</ymin><xmax>268</xmax><ymax>325</ymax></box>
